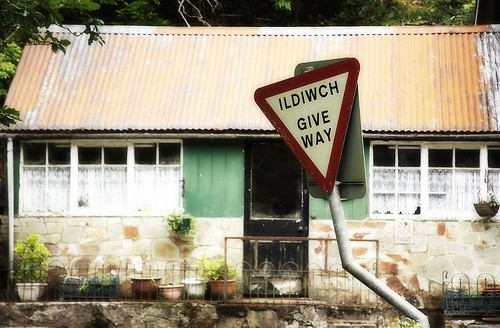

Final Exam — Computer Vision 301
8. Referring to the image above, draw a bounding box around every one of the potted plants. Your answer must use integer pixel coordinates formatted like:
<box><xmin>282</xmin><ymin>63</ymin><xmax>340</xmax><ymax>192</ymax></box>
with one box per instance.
<box><xmin>157</xmin><ymin>282</ymin><xmax>185</xmax><ymax>301</ymax></box>
<box><xmin>182</xmin><ymin>258</ymin><xmax>207</xmax><ymax>300</ymax></box>
<box><xmin>130</xmin><ymin>246</ymin><xmax>160</xmax><ymax>300</ymax></box>
<box><xmin>61</xmin><ymin>273</ymin><xmax>122</xmax><ymax>301</ymax></box>
<box><xmin>12</xmin><ymin>232</ymin><xmax>51</xmax><ymax>302</ymax></box>
<box><xmin>473</xmin><ymin>191</ymin><xmax>500</xmax><ymax>217</ymax></box>
<box><xmin>441</xmin><ymin>285</ymin><xmax>500</xmax><ymax>311</ymax></box>
<box><xmin>167</xmin><ymin>210</ymin><xmax>196</xmax><ymax>251</ymax></box>
<box><xmin>206</xmin><ymin>258</ymin><xmax>237</xmax><ymax>301</ymax></box>
